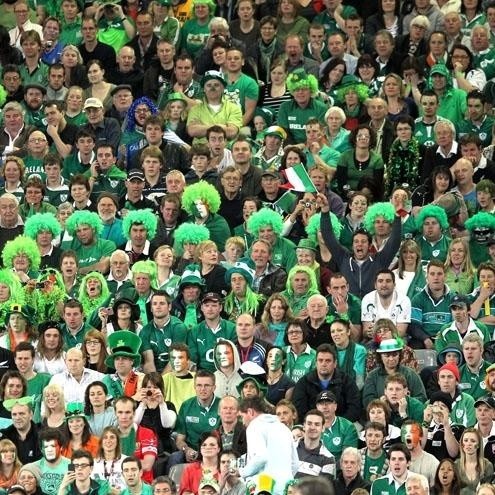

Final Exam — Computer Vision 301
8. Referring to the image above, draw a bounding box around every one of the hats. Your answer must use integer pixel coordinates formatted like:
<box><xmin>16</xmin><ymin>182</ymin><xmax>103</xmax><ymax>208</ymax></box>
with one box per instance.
<box><xmin>63</xmin><ymin>402</ymin><xmax>90</xmax><ymax>422</ymax></box>
<box><xmin>436</xmin><ymin>362</ymin><xmax>461</xmax><ymax>381</ymax></box>
<box><xmin>260</xmin><ymin>168</ymin><xmax>280</xmax><ymax>177</ymax></box>
<box><xmin>293</xmin><ymin>238</ymin><xmax>318</xmax><ymax>252</ymax></box>
<box><xmin>23</xmin><ymin>70</ymin><xmax>227</xmax><ymax>110</ymax></box>
<box><xmin>473</xmin><ymin>395</ymin><xmax>495</xmax><ymax>409</ymax></box>
<box><xmin>103</xmin><ymin>330</ymin><xmax>142</xmax><ymax>370</ymax></box>
<box><xmin>337</xmin><ymin>74</ymin><xmax>364</xmax><ymax>88</ymax></box>
<box><xmin>429</xmin><ymin>390</ymin><xmax>453</xmax><ymax>412</ymax></box>
<box><xmin>447</xmin><ymin>295</ymin><xmax>470</xmax><ymax>310</ymax></box>
<box><xmin>110</xmin><ymin>287</ymin><xmax>141</xmax><ymax>322</ymax></box>
<box><xmin>177</xmin><ymin>261</ymin><xmax>254</xmax><ymax>305</ymax></box>
<box><xmin>375</xmin><ymin>338</ymin><xmax>403</xmax><ymax>353</ymax></box>
<box><xmin>314</xmin><ymin>391</ymin><xmax>336</xmax><ymax>402</ymax></box>
<box><xmin>126</xmin><ymin>167</ymin><xmax>146</xmax><ymax>183</ymax></box>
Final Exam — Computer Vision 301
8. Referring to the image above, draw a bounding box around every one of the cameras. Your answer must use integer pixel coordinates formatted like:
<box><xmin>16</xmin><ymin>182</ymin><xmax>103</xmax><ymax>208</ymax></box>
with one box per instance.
<box><xmin>100</xmin><ymin>307</ymin><xmax>114</xmax><ymax>316</ymax></box>
<box><xmin>430</xmin><ymin>406</ymin><xmax>442</xmax><ymax>412</ymax></box>
<box><xmin>302</xmin><ymin>200</ymin><xmax>312</xmax><ymax>208</ymax></box>
<box><xmin>36</xmin><ymin>283</ymin><xmax>45</xmax><ymax>288</ymax></box>
<box><xmin>146</xmin><ymin>389</ymin><xmax>155</xmax><ymax>396</ymax></box>
<box><xmin>46</xmin><ymin>41</ymin><xmax>53</xmax><ymax>48</ymax></box>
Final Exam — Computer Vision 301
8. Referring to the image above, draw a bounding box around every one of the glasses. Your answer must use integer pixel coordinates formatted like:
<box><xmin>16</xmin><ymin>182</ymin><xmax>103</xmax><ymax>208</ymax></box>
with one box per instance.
<box><xmin>73</xmin><ymin>463</ymin><xmax>90</xmax><ymax>469</ymax></box>
<box><xmin>27</xmin><ymin>138</ymin><xmax>46</xmax><ymax>143</ymax></box>
<box><xmin>411</xmin><ymin>24</ymin><xmax>428</xmax><ymax>31</ymax></box>
<box><xmin>451</xmin><ymin>53</ymin><xmax>469</xmax><ymax>60</ymax></box>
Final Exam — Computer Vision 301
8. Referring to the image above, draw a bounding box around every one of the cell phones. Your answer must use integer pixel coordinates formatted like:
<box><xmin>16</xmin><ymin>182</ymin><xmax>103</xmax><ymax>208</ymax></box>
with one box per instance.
<box><xmin>451</xmin><ymin>190</ymin><xmax>457</xmax><ymax>199</ymax></box>
<box><xmin>68</xmin><ymin>464</ymin><xmax>75</xmax><ymax>474</ymax></box>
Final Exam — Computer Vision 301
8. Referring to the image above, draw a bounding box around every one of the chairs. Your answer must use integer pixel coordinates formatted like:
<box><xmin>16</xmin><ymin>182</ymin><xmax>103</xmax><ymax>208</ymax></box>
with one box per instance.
<box><xmin>414</xmin><ymin>350</ymin><xmax>437</xmax><ymax>374</ymax></box>
